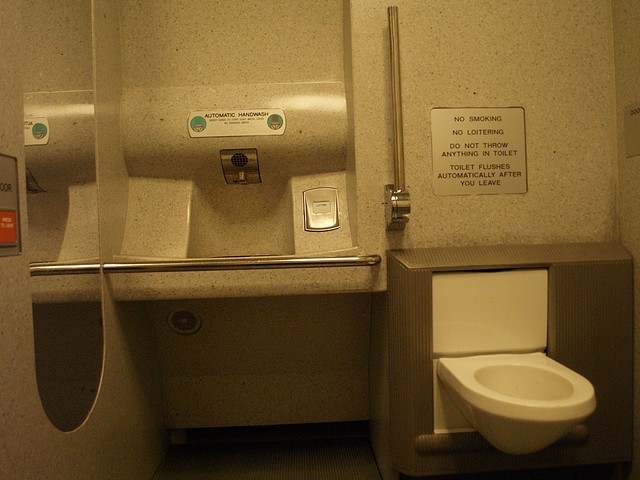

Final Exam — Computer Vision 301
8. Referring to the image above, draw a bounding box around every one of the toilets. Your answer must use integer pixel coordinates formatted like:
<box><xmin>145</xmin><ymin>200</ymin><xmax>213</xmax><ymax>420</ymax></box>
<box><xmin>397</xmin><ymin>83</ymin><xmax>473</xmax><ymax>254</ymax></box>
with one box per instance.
<box><xmin>433</xmin><ymin>269</ymin><xmax>597</xmax><ymax>454</ymax></box>
